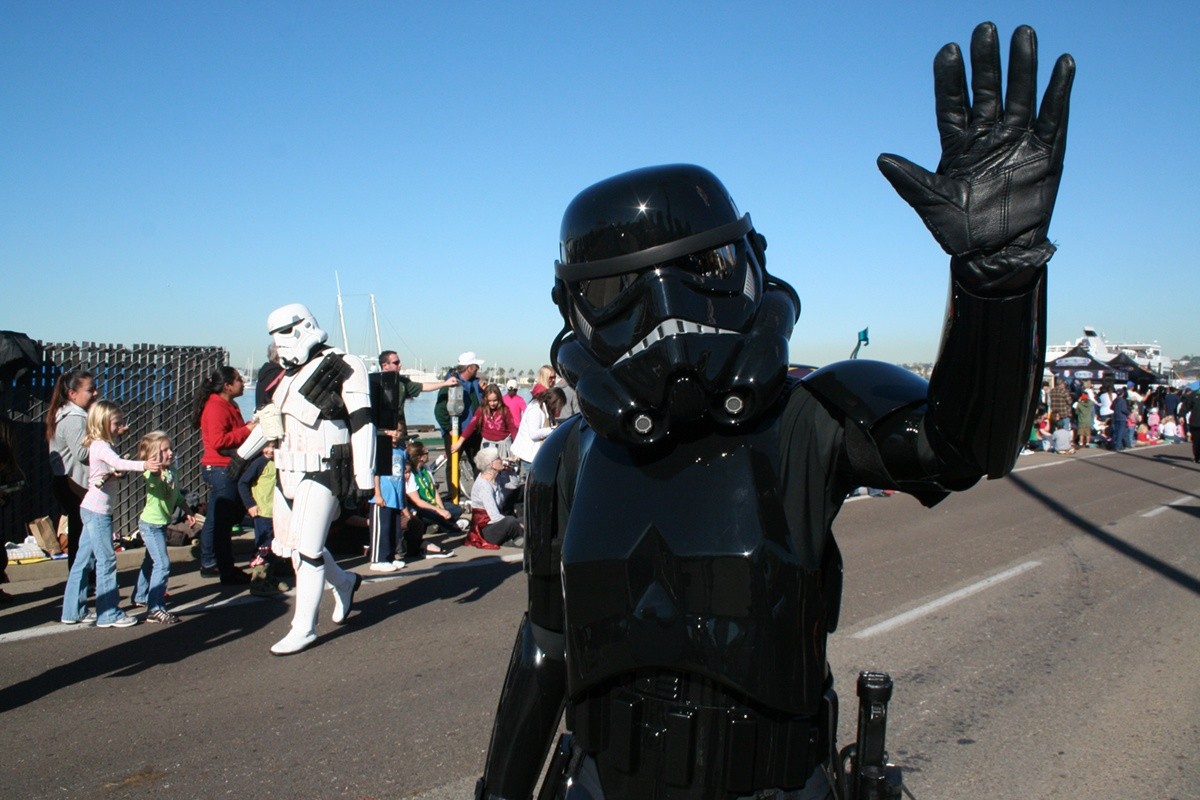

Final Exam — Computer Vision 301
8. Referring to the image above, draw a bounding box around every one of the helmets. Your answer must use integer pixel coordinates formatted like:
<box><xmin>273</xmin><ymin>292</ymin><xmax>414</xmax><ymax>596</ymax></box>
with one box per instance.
<box><xmin>267</xmin><ymin>303</ymin><xmax>329</xmax><ymax>369</ymax></box>
<box><xmin>549</xmin><ymin>163</ymin><xmax>801</xmax><ymax>454</ymax></box>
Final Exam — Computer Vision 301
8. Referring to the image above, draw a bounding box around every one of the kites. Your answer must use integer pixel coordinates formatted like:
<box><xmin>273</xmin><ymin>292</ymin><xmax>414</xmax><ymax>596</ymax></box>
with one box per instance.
<box><xmin>849</xmin><ymin>326</ymin><xmax>869</xmax><ymax>360</ymax></box>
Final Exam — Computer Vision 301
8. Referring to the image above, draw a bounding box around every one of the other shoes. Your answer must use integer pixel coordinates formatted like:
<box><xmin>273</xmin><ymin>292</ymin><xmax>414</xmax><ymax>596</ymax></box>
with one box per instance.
<box><xmin>269</xmin><ymin>627</ymin><xmax>321</xmax><ymax>655</ymax></box>
<box><xmin>420</xmin><ymin>539</ymin><xmax>454</xmax><ymax>558</ymax></box>
<box><xmin>332</xmin><ymin>572</ymin><xmax>363</xmax><ymax>625</ymax></box>
<box><xmin>200</xmin><ymin>560</ymin><xmax>251</xmax><ymax>586</ymax></box>
<box><xmin>370</xmin><ymin>560</ymin><xmax>407</xmax><ymax>571</ymax></box>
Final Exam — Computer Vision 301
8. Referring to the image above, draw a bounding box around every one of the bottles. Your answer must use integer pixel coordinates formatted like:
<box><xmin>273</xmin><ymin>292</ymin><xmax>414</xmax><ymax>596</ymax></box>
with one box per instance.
<box><xmin>501</xmin><ymin>460</ymin><xmax>519</xmax><ymax>472</ymax></box>
<box><xmin>430</xmin><ymin>453</ymin><xmax>446</xmax><ymax>469</ymax></box>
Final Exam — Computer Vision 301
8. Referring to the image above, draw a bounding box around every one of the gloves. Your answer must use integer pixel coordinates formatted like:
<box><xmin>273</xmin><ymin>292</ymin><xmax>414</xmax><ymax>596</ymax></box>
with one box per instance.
<box><xmin>877</xmin><ymin>21</ymin><xmax>1076</xmax><ymax>292</ymax></box>
<box><xmin>225</xmin><ymin>450</ymin><xmax>245</xmax><ymax>479</ymax></box>
<box><xmin>354</xmin><ymin>487</ymin><xmax>375</xmax><ymax>500</ymax></box>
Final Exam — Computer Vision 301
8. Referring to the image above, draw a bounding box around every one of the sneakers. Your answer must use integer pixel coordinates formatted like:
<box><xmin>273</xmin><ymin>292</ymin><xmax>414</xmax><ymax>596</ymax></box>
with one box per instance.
<box><xmin>132</xmin><ymin>599</ymin><xmax>147</xmax><ymax>608</ymax></box>
<box><xmin>146</xmin><ymin>610</ymin><xmax>180</xmax><ymax>625</ymax></box>
<box><xmin>62</xmin><ymin>608</ymin><xmax>139</xmax><ymax>627</ymax></box>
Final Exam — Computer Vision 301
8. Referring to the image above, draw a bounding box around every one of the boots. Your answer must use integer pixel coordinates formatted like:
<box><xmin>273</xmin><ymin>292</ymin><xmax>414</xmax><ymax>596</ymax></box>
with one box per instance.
<box><xmin>250</xmin><ymin>563</ymin><xmax>290</xmax><ymax>596</ymax></box>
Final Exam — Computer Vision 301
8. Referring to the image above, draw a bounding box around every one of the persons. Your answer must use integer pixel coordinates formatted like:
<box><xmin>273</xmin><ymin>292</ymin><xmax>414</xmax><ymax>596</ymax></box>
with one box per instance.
<box><xmin>255</xmin><ymin>344</ymin><xmax>284</xmax><ymax>408</ymax></box>
<box><xmin>369</xmin><ymin>346</ymin><xmax>580</xmax><ymax>573</ymax></box>
<box><xmin>130</xmin><ymin>431</ymin><xmax>196</xmax><ymax>623</ymax></box>
<box><xmin>47</xmin><ymin>370</ymin><xmax>98</xmax><ymax>599</ymax></box>
<box><xmin>1016</xmin><ymin>368</ymin><xmax>1199</xmax><ymax>463</ymax></box>
<box><xmin>192</xmin><ymin>366</ymin><xmax>259</xmax><ymax>586</ymax></box>
<box><xmin>476</xmin><ymin>18</ymin><xmax>1074</xmax><ymax>800</ymax></box>
<box><xmin>224</xmin><ymin>302</ymin><xmax>375</xmax><ymax>654</ymax></box>
<box><xmin>238</xmin><ymin>438</ymin><xmax>281</xmax><ymax>599</ymax></box>
<box><xmin>60</xmin><ymin>400</ymin><xmax>162</xmax><ymax>630</ymax></box>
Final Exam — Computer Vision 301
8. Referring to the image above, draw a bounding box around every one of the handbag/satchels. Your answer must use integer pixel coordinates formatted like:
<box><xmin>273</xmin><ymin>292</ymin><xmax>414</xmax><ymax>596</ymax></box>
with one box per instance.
<box><xmin>28</xmin><ymin>515</ymin><xmax>63</xmax><ymax>557</ymax></box>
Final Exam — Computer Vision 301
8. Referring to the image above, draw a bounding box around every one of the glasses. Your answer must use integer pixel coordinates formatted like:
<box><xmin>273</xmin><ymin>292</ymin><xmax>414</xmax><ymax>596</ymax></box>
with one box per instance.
<box><xmin>419</xmin><ymin>450</ymin><xmax>430</xmax><ymax>456</ymax></box>
<box><xmin>390</xmin><ymin>360</ymin><xmax>400</xmax><ymax>365</ymax></box>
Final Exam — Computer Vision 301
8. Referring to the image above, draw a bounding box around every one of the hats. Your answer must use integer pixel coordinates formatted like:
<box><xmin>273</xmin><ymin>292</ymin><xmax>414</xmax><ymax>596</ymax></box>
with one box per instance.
<box><xmin>456</xmin><ymin>352</ymin><xmax>485</xmax><ymax>366</ymax></box>
<box><xmin>472</xmin><ymin>447</ymin><xmax>500</xmax><ymax>471</ymax></box>
<box><xmin>506</xmin><ymin>379</ymin><xmax>521</xmax><ymax>391</ymax></box>
